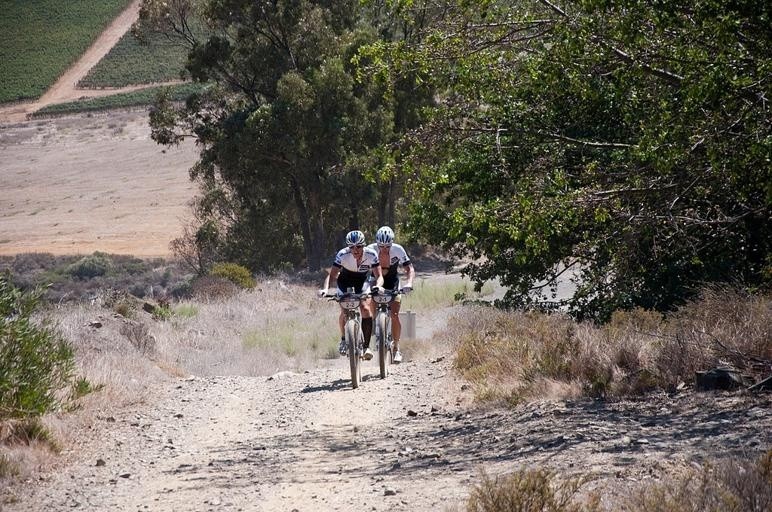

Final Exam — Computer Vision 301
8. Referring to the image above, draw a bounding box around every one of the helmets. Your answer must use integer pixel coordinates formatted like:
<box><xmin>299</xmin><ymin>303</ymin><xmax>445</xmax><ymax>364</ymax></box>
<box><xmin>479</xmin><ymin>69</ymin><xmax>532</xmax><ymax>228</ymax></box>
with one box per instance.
<box><xmin>375</xmin><ymin>225</ymin><xmax>395</xmax><ymax>247</ymax></box>
<box><xmin>345</xmin><ymin>230</ymin><xmax>367</xmax><ymax>248</ymax></box>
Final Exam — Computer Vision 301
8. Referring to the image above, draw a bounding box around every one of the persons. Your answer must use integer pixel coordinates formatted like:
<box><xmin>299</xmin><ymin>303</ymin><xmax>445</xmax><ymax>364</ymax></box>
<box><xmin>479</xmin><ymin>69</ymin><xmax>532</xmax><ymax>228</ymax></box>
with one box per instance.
<box><xmin>366</xmin><ymin>225</ymin><xmax>416</xmax><ymax>363</ymax></box>
<box><xmin>315</xmin><ymin>229</ymin><xmax>384</xmax><ymax>361</ymax></box>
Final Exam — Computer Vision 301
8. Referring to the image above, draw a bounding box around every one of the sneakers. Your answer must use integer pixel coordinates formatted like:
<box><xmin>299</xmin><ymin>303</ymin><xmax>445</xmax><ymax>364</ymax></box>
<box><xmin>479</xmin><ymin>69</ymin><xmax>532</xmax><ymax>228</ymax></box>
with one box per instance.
<box><xmin>363</xmin><ymin>349</ymin><xmax>374</xmax><ymax>360</ymax></box>
<box><xmin>339</xmin><ymin>341</ymin><xmax>348</xmax><ymax>356</ymax></box>
<box><xmin>393</xmin><ymin>350</ymin><xmax>402</xmax><ymax>363</ymax></box>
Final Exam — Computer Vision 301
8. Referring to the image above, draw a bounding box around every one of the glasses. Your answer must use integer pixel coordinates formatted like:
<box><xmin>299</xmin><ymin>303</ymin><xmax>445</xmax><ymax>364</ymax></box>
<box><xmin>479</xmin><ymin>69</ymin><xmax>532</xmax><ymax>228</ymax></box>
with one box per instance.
<box><xmin>378</xmin><ymin>245</ymin><xmax>391</xmax><ymax>249</ymax></box>
<box><xmin>348</xmin><ymin>245</ymin><xmax>362</xmax><ymax>249</ymax></box>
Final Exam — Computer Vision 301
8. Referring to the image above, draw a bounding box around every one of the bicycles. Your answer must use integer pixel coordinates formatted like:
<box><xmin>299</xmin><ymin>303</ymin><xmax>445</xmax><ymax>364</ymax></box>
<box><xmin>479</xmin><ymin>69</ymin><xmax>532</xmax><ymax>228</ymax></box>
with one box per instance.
<box><xmin>322</xmin><ymin>286</ymin><xmax>413</xmax><ymax>389</ymax></box>
